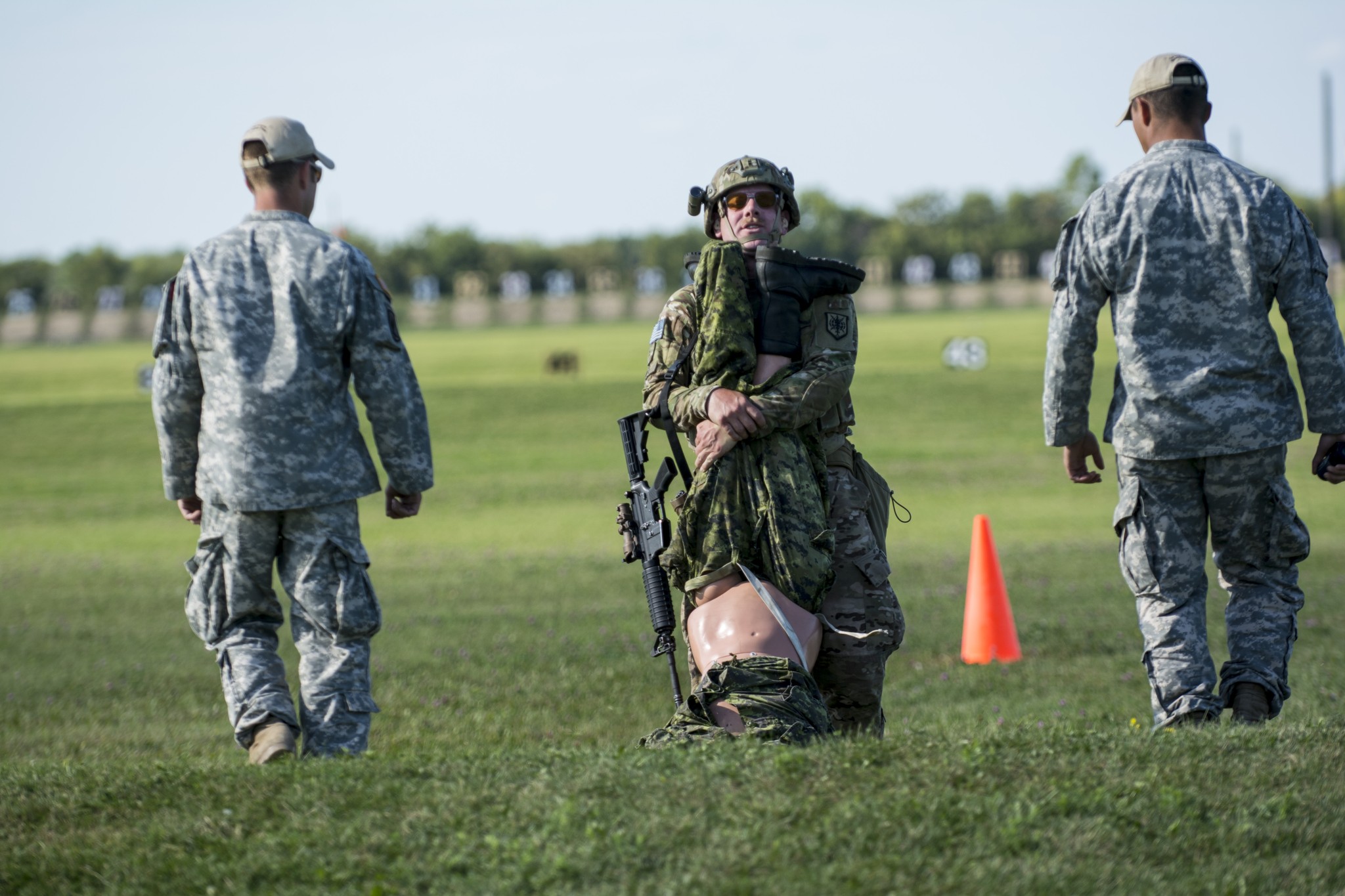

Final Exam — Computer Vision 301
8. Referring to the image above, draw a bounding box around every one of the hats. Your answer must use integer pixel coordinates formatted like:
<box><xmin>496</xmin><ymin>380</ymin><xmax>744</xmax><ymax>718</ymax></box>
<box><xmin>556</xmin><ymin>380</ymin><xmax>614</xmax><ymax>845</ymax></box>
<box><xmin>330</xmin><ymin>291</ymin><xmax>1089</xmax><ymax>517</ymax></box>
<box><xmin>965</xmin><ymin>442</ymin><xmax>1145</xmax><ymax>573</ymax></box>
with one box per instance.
<box><xmin>1115</xmin><ymin>54</ymin><xmax>1209</xmax><ymax>129</ymax></box>
<box><xmin>240</xmin><ymin>115</ymin><xmax>335</xmax><ymax>169</ymax></box>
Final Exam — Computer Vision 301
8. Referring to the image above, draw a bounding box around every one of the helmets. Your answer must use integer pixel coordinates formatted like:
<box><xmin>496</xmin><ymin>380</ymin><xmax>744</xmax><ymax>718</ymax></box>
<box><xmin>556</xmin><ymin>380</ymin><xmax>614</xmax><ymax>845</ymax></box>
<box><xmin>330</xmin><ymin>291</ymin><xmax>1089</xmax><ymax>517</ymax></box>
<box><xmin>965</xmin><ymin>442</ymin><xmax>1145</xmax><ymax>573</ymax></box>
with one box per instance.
<box><xmin>703</xmin><ymin>156</ymin><xmax>800</xmax><ymax>241</ymax></box>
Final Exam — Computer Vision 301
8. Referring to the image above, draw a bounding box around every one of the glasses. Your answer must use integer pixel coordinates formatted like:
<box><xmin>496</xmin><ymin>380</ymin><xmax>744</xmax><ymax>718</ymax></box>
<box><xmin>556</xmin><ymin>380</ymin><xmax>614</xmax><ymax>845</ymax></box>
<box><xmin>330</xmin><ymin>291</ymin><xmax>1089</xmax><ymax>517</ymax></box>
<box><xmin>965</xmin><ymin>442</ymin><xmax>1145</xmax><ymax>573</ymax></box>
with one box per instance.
<box><xmin>290</xmin><ymin>156</ymin><xmax>323</xmax><ymax>181</ymax></box>
<box><xmin>721</xmin><ymin>190</ymin><xmax>780</xmax><ymax>210</ymax></box>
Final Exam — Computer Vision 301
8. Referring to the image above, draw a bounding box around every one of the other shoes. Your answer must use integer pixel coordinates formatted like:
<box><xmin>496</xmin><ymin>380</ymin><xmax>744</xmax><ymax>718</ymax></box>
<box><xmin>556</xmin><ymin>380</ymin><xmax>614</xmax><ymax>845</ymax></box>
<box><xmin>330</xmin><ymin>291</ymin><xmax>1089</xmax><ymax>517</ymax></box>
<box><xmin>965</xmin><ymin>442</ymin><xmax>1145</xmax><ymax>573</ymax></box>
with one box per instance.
<box><xmin>247</xmin><ymin>718</ymin><xmax>297</xmax><ymax>766</ymax></box>
<box><xmin>1230</xmin><ymin>684</ymin><xmax>1270</xmax><ymax>729</ymax></box>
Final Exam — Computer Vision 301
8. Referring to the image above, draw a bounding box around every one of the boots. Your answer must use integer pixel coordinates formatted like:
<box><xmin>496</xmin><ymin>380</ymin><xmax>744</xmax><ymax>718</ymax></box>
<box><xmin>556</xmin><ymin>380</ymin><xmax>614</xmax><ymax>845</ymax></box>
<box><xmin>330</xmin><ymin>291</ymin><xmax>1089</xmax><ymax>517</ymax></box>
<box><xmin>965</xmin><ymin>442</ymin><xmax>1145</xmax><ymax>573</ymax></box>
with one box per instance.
<box><xmin>683</xmin><ymin>252</ymin><xmax>701</xmax><ymax>279</ymax></box>
<box><xmin>753</xmin><ymin>244</ymin><xmax>865</xmax><ymax>360</ymax></box>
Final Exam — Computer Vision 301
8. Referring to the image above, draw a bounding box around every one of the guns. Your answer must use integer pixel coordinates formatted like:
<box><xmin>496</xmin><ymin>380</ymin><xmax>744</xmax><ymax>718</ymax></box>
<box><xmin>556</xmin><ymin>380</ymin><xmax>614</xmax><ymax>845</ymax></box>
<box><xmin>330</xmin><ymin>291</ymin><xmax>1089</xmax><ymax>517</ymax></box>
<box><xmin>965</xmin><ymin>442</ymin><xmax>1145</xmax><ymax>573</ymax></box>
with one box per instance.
<box><xmin>609</xmin><ymin>404</ymin><xmax>682</xmax><ymax>703</ymax></box>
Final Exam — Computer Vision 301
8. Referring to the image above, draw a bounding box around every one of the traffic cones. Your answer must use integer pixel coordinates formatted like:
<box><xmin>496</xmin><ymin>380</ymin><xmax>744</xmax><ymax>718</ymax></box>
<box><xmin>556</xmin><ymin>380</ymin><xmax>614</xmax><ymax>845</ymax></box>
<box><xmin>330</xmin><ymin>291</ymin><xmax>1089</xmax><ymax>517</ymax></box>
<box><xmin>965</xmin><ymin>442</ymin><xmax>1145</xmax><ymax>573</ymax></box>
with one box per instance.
<box><xmin>961</xmin><ymin>511</ymin><xmax>1026</xmax><ymax>666</ymax></box>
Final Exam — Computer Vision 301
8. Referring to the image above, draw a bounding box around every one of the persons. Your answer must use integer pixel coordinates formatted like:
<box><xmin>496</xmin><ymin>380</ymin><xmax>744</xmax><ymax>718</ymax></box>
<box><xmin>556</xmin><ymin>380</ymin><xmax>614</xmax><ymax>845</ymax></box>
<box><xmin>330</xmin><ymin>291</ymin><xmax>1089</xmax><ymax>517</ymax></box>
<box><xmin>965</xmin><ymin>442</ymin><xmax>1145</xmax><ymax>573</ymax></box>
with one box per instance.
<box><xmin>151</xmin><ymin>116</ymin><xmax>435</xmax><ymax>768</ymax></box>
<box><xmin>640</xmin><ymin>157</ymin><xmax>906</xmax><ymax>741</ymax></box>
<box><xmin>1042</xmin><ymin>53</ymin><xmax>1345</xmax><ymax>728</ymax></box>
